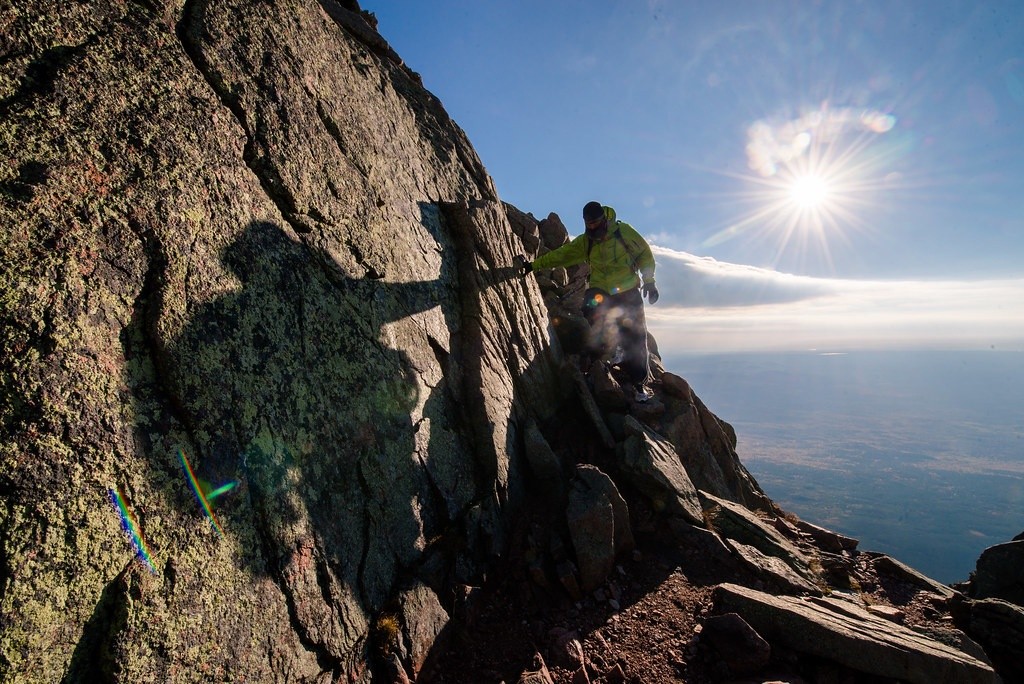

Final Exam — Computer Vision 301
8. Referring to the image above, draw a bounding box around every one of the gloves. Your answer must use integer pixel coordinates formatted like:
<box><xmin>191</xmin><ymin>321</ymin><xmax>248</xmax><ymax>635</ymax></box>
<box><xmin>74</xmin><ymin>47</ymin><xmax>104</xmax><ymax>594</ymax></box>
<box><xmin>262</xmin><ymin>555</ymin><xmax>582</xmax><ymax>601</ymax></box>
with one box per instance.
<box><xmin>643</xmin><ymin>281</ymin><xmax>658</xmax><ymax>303</ymax></box>
<box><xmin>517</xmin><ymin>254</ymin><xmax>534</xmax><ymax>277</ymax></box>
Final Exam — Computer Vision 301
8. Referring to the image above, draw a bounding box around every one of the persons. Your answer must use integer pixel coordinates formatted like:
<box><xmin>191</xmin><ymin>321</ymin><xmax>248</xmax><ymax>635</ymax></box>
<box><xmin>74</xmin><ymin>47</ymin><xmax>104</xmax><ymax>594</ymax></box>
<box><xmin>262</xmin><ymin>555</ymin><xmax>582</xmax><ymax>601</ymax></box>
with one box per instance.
<box><xmin>518</xmin><ymin>202</ymin><xmax>660</xmax><ymax>387</ymax></box>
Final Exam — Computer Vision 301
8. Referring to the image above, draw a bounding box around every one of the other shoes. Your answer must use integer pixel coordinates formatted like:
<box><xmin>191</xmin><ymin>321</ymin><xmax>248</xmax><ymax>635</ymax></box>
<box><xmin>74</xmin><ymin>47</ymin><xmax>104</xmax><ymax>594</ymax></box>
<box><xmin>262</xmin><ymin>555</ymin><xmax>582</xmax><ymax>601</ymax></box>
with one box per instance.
<box><xmin>631</xmin><ymin>364</ymin><xmax>647</xmax><ymax>386</ymax></box>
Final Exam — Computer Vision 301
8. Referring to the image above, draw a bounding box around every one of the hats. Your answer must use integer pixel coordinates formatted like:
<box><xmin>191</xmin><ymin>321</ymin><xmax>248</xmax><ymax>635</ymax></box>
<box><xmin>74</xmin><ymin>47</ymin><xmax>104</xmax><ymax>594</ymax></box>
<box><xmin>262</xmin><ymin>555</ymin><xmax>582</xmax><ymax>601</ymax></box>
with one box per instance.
<box><xmin>583</xmin><ymin>202</ymin><xmax>603</xmax><ymax>221</ymax></box>
